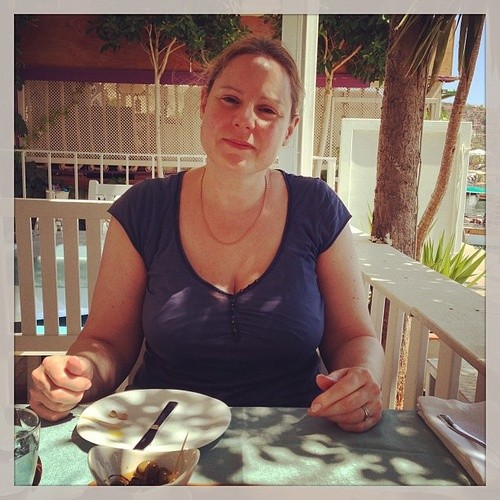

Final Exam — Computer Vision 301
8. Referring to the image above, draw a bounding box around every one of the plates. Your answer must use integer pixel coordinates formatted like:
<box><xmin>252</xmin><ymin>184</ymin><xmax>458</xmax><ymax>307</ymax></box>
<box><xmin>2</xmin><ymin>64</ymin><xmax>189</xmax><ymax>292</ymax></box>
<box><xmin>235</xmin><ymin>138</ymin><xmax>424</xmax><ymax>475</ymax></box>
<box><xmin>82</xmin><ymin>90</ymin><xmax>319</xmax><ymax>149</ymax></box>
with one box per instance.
<box><xmin>77</xmin><ymin>389</ymin><xmax>232</xmax><ymax>452</ymax></box>
<box><xmin>88</xmin><ymin>445</ymin><xmax>201</xmax><ymax>486</ymax></box>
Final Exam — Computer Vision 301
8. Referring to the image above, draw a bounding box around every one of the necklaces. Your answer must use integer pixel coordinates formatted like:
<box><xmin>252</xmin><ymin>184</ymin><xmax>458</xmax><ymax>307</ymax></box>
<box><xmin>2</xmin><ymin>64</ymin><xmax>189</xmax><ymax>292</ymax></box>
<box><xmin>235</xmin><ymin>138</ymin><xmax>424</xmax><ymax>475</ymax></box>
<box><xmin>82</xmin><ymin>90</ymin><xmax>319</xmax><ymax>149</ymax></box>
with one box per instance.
<box><xmin>200</xmin><ymin>164</ymin><xmax>268</xmax><ymax>246</ymax></box>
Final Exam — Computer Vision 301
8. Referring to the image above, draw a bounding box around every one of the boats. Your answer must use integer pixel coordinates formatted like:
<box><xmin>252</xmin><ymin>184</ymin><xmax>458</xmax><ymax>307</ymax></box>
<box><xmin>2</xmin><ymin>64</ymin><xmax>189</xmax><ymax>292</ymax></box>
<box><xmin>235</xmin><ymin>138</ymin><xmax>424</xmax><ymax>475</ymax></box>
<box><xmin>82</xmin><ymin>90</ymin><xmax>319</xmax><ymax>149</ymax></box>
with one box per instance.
<box><xmin>463</xmin><ymin>185</ymin><xmax>486</xmax><ymax>246</ymax></box>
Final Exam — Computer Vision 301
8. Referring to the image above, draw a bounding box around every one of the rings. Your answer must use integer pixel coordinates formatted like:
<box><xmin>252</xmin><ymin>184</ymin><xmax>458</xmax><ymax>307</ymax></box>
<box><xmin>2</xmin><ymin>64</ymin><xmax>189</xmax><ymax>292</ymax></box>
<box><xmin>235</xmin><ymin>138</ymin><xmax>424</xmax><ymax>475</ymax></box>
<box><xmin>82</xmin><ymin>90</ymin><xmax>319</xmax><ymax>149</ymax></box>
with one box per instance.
<box><xmin>360</xmin><ymin>404</ymin><xmax>373</xmax><ymax>418</ymax></box>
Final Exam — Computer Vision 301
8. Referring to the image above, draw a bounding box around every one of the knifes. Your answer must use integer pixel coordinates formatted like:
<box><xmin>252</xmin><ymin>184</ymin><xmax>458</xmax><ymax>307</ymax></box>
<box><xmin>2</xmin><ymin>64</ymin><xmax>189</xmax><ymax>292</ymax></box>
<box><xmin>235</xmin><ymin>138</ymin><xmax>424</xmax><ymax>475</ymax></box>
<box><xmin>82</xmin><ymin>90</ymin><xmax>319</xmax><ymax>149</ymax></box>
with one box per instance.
<box><xmin>134</xmin><ymin>401</ymin><xmax>178</xmax><ymax>450</ymax></box>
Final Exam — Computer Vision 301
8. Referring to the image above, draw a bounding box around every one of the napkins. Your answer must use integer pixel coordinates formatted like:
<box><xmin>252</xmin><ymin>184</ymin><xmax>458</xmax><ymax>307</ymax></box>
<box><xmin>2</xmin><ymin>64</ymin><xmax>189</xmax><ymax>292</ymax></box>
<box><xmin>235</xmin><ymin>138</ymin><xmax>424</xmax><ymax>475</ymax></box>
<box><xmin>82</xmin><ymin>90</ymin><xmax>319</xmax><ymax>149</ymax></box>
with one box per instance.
<box><xmin>418</xmin><ymin>396</ymin><xmax>486</xmax><ymax>486</ymax></box>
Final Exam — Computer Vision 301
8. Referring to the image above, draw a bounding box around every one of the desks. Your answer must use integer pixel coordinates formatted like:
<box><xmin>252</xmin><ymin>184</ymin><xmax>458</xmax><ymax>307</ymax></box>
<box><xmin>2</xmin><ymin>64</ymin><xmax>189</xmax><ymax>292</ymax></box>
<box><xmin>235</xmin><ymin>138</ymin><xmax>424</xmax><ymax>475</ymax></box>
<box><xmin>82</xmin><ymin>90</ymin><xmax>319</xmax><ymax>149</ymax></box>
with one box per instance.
<box><xmin>15</xmin><ymin>404</ymin><xmax>476</xmax><ymax>486</ymax></box>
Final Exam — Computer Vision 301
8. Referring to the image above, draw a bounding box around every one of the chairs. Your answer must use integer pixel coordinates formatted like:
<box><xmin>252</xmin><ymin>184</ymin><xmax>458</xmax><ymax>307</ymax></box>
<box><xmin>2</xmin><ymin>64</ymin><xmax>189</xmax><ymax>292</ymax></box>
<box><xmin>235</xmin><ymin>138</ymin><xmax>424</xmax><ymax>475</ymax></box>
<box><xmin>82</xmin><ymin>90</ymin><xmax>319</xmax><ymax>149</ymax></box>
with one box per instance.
<box><xmin>14</xmin><ymin>180</ymin><xmax>136</xmax><ymax>404</ymax></box>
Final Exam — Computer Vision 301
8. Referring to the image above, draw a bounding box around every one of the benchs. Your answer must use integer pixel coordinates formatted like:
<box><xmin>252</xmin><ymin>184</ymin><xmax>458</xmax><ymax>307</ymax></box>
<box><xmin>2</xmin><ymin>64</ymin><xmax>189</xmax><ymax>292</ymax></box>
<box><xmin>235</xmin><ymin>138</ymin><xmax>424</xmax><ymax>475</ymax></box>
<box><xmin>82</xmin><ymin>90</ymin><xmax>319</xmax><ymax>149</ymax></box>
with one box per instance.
<box><xmin>425</xmin><ymin>357</ymin><xmax>478</xmax><ymax>404</ymax></box>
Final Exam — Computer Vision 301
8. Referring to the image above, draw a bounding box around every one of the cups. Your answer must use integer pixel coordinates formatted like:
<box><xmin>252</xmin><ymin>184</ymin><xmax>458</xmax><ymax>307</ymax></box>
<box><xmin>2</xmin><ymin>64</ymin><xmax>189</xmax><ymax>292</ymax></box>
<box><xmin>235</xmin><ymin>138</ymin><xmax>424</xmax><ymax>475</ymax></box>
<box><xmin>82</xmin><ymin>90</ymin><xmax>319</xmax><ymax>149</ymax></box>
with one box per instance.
<box><xmin>14</xmin><ymin>408</ymin><xmax>40</xmax><ymax>485</ymax></box>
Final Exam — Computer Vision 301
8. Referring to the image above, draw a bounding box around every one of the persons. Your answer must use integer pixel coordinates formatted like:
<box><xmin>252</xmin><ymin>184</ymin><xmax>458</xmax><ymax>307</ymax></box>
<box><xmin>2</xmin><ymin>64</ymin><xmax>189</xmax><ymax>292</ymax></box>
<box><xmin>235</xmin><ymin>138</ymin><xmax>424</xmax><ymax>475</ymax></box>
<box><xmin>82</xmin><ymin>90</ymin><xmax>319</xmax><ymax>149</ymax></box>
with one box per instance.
<box><xmin>28</xmin><ymin>34</ymin><xmax>386</xmax><ymax>433</ymax></box>
